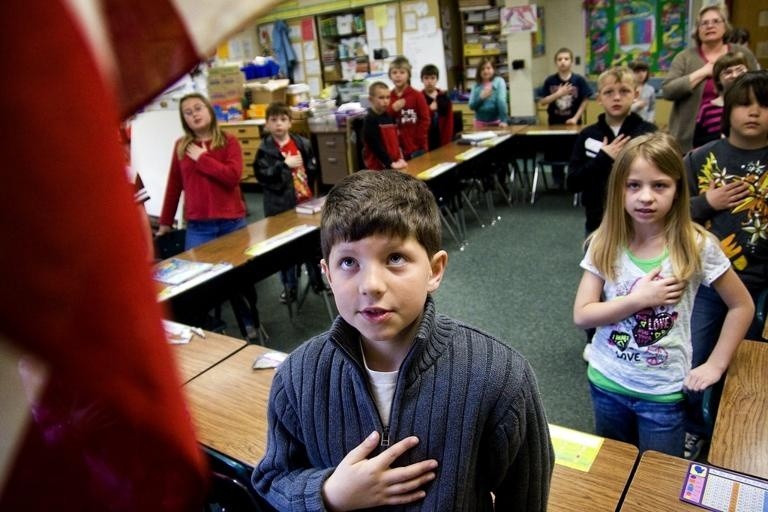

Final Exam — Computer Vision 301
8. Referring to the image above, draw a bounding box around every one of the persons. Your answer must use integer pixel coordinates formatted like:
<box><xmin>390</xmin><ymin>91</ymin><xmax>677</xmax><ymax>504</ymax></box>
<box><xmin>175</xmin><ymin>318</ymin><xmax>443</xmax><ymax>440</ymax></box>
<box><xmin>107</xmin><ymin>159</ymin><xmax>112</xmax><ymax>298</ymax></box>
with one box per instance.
<box><xmin>573</xmin><ymin>129</ymin><xmax>753</xmax><ymax>456</ymax></box>
<box><xmin>694</xmin><ymin>49</ymin><xmax>750</xmax><ymax>153</ymax></box>
<box><xmin>251</xmin><ymin>102</ymin><xmax>333</xmax><ymax>302</ymax></box>
<box><xmin>468</xmin><ymin>57</ymin><xmax>510</xmax><ymax>198</ymax></box>
<box><xmin>570</xmin><ymin>65</ymin><xmax>659</xmax><ymax>370</ymax></box>
<box><xmin>631</xmin><ymin>62</ymin><xmax>656</xmax><ymax>124</ymax></box>
<box><xmin>156</xmin><ymin>93</ymin><xmax>261</xmax><ymax>333</ymax></box>
<box><xmin>354</xmin><ymin>55</ymin><xmax>453</xmax><ymax>171</ymax></box>
<box><xmin>681</xmin><ymin>68</ymin><xmax>768</xmax><ymax>466</ymax></box>
<box><xmin>538</xmin><ymin>49</ymin><xmax>593</xmax><ymax>195</ymax></box>
<box><xmin>724</xmin><ymin>28</ymin><xmax>751</xmax><ymax>48</ymax></box>
<box><xmin>663</xmin><ymin>2</ymin><xmax>760</xmax><ymax>160</ymax></box>
<box><xmin>251</xmin><ymin>169</ymin><xmax>555</xmax><ymax>511</ymax></box>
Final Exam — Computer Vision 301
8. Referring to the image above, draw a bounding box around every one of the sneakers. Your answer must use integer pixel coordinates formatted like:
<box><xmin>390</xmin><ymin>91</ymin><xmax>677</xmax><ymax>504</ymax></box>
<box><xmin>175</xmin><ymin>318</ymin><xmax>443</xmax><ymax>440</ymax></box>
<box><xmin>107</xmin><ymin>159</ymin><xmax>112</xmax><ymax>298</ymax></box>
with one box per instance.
<box><xmin>684</xmin><ymin>432</ymin><xmax>703</xmax><ymax>460</ymax></box>
<box><xmin>279</xmin><ymin>288</ymin><xmax>297</xmax><ymax>302</ymax></box>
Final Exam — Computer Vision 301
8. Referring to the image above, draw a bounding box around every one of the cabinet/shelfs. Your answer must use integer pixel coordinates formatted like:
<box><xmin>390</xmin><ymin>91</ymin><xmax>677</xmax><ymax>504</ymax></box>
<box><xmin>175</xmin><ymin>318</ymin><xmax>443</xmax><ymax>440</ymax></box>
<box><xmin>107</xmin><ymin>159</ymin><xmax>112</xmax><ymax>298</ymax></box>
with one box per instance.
<box><xmin>220</xmin><ymin>110</ymin><xmax>367</xmax><ymax>184</ymax></box>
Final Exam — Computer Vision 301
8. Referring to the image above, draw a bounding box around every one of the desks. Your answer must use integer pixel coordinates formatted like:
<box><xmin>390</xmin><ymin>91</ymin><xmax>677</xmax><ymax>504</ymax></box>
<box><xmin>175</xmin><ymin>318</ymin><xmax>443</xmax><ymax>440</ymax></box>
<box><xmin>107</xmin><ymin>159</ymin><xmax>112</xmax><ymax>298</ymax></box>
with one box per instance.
<box><xmin>617</xmin><ymin>450</ymin><xmax>768</xmax><ymax>511</ymax></box>
<box><xmin>706</xmin><ymin>324</ymin><xmax>768</xmax><ymax>482</ymax></box>
<box><xmin>759</xmin><ymin>314</ymin><xmax>768</xmax><ymax>344</ymax></box>
<box><xmin>152</xmin><ymin>123</ymin><xmax>527</xmax><ymax>319</ymax></box>
<box><xmin>507</xmin><ymin>122</ymin><xmax>581</xmax><ymax>207</ymax></box>
<box><xmin>180</xmin><ymin>343</ymin><xmax>642</xmax><ymax>512</ymax></box>
<box><xmin>159</xmin><ymin>318</ymin><xmax>249</xmax><ymax>388</ymax></box>
